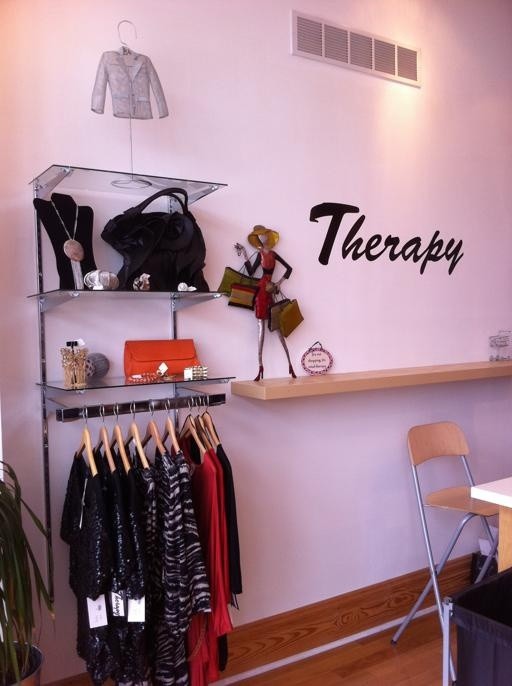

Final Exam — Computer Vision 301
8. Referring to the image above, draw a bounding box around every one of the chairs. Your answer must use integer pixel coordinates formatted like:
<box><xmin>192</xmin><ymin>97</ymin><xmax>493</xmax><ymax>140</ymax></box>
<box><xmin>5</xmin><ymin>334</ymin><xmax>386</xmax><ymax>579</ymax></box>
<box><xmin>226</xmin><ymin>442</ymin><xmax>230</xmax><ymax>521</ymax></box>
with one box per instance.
<box><xmin>391</xmin><ymin>418</ymin><xmax>497</xmax><ymax>686</ymax></box>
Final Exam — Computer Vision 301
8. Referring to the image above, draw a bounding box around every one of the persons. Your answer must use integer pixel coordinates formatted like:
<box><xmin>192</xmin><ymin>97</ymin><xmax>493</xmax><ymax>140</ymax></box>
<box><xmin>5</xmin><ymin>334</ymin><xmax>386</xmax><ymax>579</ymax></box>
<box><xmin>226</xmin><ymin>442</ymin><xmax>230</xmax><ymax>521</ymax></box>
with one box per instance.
<box><xmin>233</xmin><ymin>226</ymin><xmax>297</xmax><ymax>382</ymax></box>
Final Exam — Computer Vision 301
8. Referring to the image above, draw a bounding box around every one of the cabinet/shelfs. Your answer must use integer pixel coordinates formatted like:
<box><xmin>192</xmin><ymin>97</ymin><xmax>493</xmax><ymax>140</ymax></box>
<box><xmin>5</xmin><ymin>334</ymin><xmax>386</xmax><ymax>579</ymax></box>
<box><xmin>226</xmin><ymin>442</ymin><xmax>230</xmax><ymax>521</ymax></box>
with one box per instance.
<box><xmin>27</xmin><ymin>163</ymin><xmax>237</xmax><ymax>609</ymax></box>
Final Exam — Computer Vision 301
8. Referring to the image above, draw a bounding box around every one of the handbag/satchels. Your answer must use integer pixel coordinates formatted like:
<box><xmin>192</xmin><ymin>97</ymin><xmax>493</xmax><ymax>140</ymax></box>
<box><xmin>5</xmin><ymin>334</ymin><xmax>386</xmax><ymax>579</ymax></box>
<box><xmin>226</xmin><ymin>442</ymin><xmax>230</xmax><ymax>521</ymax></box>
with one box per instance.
<box><xmin>101</xmin><ymin>187</ymin><xmax>209</xmax><ymax>292</ymax></box>
<box><xmin>124</xmin><ymin>339</ymin><xmax>199</xmax><ymax>385</ymax></box>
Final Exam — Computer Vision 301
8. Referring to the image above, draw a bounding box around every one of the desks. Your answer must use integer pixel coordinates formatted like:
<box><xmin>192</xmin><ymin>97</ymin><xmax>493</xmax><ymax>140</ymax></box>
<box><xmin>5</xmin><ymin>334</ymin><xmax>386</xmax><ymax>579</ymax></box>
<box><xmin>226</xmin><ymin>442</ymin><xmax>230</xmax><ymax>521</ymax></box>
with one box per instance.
<box><xmin>469</xmin><ymin>473</ymin><xmax>511</xmax><ymax>576</ymax></box>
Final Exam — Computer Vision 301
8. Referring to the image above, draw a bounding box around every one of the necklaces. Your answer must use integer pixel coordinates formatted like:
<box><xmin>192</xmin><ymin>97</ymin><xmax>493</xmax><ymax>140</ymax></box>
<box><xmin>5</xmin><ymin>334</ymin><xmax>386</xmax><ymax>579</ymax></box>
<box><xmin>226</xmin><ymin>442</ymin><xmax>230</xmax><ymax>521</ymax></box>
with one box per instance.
<box><xmin>51</xmin><ymin>200</ymin><xmax>85</xmax><ymax>261</ymax></box>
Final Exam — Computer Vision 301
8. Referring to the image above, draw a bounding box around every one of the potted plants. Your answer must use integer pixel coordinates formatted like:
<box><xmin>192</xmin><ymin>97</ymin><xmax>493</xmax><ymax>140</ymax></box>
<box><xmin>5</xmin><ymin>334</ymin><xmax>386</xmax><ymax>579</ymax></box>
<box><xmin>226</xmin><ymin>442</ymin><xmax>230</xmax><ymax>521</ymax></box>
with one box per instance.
<box><xmin>2</xmin><ymin>462</ymin><xmax>45</xmax><ymax>685</ymax></box>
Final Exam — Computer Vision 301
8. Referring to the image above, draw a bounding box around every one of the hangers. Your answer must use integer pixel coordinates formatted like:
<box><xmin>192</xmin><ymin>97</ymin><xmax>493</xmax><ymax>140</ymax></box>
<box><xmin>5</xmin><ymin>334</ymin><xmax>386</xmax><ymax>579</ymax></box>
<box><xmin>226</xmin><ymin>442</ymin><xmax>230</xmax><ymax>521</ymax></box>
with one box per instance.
<box><xmin>72</xmin><ymin>393</ymin><xmax>223</xmax><ymax>477</ymax></box>
